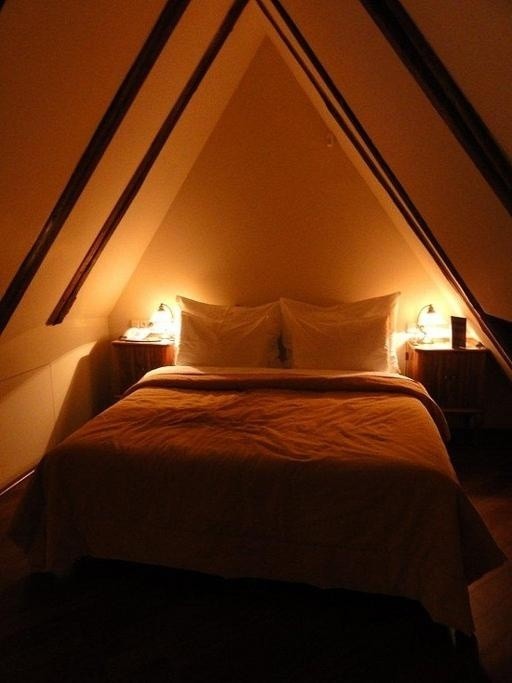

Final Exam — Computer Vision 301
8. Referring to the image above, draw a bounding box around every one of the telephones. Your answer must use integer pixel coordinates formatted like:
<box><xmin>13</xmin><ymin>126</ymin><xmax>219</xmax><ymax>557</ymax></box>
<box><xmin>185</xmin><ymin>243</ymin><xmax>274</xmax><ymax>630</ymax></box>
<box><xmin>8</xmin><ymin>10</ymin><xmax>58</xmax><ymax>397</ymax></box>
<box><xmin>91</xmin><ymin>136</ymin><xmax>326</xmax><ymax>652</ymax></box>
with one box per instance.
<box><xmin>121</xmin><ymin>327</ymin><xmax>151</xmax><ymax>341</ymax></box>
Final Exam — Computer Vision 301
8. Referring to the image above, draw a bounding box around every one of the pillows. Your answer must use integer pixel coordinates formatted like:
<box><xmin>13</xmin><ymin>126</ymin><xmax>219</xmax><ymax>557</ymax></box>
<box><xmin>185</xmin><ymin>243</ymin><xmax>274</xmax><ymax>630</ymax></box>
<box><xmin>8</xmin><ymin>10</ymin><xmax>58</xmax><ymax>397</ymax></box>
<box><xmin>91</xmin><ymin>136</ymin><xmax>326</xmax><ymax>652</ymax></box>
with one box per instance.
<box><xmin>172</xmin><ymin>281</ymin><xmax>403</xmax><ymax>375</ymax></box>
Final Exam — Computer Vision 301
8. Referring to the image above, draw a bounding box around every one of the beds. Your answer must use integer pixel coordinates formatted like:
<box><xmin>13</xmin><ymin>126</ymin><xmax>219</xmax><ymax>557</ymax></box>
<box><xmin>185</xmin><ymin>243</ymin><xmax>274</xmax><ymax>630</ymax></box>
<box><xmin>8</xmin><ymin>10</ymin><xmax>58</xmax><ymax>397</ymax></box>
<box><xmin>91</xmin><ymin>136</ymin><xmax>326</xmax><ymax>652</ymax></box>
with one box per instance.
<box><xmin>3</xmin><ymin>359</ymin><xmax>508</xmax><ymax>658</ymax></box>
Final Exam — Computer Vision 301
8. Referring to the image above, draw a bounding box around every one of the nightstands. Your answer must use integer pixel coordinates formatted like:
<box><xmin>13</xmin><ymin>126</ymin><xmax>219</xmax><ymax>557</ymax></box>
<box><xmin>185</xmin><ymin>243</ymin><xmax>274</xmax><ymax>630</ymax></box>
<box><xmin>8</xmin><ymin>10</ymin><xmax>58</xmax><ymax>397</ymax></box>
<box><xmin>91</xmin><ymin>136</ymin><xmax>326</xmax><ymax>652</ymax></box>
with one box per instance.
<box><xmin>405</xmin><ymin>337</ymin><xmax>489</xmax><ymax>446</ymax></box>
<box><xmin>109</xmin><ymin>337</ymin><xmax>177</xmax><ymax>406</ymax></box>
